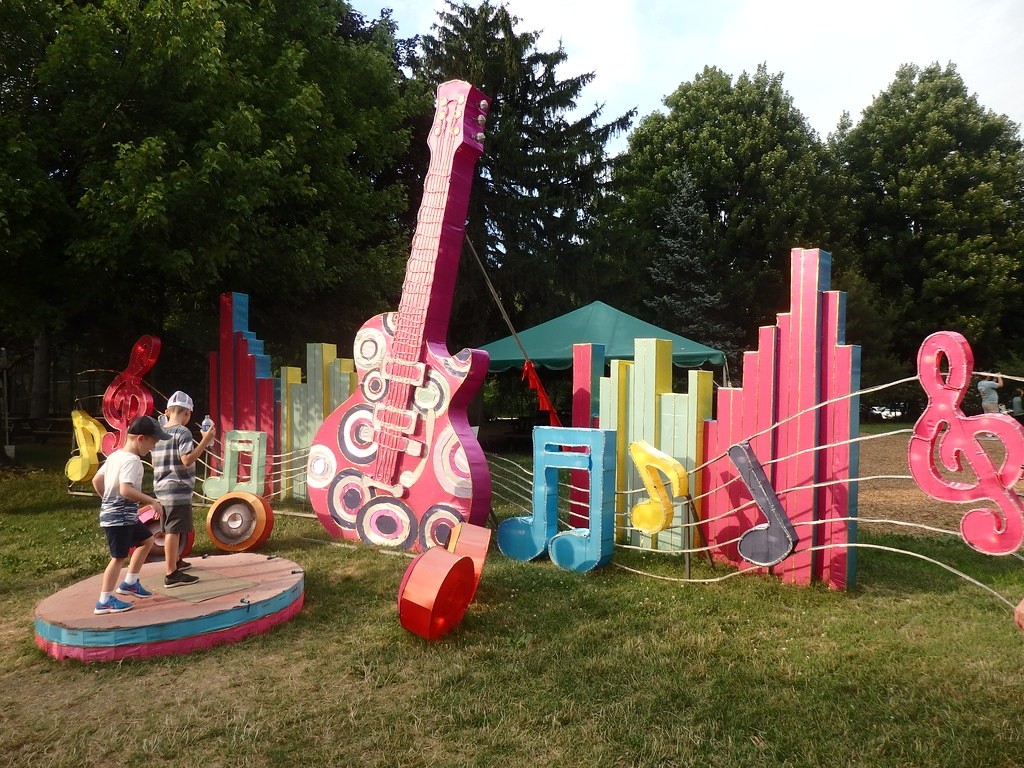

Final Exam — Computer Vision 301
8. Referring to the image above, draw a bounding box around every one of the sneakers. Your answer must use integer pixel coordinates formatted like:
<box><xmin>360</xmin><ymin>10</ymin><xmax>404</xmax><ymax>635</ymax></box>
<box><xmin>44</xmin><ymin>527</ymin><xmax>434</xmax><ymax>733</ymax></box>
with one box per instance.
<box><xmin>165</xmin><ymin>558</ymin><xmax>199</xmax><ymax>588</ymax></box>
<box><xmin>116</xmin><ymin>578</ymin><xmax>152</xmax><ymax>598</ymax></box>
<box><xmin>94</xmin><ymin>595</ymin><xmax>133</xmax><ymax>615</ymax></box>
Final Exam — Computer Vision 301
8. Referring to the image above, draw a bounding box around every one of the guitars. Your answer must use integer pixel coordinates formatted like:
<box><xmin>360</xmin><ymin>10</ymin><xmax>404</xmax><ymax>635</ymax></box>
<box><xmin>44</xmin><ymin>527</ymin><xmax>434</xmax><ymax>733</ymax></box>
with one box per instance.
<box><xmin>307</xmin><ymin>80</ymin><xmax>491</xmax><ymax>555</ymax></box>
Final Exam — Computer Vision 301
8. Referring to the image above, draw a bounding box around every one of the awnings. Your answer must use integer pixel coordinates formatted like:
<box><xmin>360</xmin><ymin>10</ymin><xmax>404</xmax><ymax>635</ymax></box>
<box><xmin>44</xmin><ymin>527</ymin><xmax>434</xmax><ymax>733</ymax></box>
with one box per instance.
<box><xmin>454</xmin><ymin>300</ymin><xmax>726</xmax><ymax>381</ymax></box>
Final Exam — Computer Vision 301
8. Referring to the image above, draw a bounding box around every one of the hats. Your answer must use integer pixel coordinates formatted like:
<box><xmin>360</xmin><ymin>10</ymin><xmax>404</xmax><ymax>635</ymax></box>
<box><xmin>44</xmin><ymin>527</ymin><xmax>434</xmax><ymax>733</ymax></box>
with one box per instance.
<box><xmin>166</xmin><ymin>390</ymin><xmax>194</xmax><ymax>412</ymax></box>
<box><xmin>127</xmin><ymin>416</ymin><xmax>173</xmax><ymax>440</ymax></box>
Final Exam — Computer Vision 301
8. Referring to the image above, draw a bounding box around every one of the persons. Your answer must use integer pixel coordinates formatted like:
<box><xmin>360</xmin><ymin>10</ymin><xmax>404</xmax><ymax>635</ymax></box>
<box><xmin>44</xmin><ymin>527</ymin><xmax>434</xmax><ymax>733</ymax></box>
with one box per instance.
<box><xmin>1012</xmin><ymin>388</ymin><xmax>1024</xmax><ymax>428</ymax></box>
<box><xmin>90</xmin><ymin>417</ymin><xmax>174</xmax><ymax>615</ymax></box>
<box><xmin>977</xmin><ymin>370</ymin><xmax>1003</xmax><ymax>439</ymax></box>
<box><xmin>148</xmin><ymin>390</ymin><xmax>217</xmax><ymax>588</ymax></box>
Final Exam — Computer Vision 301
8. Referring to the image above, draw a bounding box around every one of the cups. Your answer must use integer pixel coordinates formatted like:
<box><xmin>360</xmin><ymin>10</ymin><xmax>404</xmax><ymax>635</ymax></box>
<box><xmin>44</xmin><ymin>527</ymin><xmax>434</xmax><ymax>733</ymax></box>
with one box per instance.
<box><xmin>158</xmin><ymin>414</ymin><xmax>168</xmax><ymax>426</ymax></box>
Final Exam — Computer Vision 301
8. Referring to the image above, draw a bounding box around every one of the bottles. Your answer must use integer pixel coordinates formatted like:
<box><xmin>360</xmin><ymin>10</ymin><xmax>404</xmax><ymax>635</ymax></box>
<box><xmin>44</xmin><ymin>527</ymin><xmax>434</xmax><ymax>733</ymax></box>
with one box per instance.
<box><xmin>202</xmin><ymin>415</ymin><xmax>214</xmax><ymax>447</ymax></box>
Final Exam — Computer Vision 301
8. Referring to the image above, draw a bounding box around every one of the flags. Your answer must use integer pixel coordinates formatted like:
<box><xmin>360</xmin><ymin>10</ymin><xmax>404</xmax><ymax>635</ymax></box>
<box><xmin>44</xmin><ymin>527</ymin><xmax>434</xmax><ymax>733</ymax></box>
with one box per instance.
<box><xmin>520</xmin><ymin>358</ymin><xmax>570</xmax><ymax>452</ymax></box>
